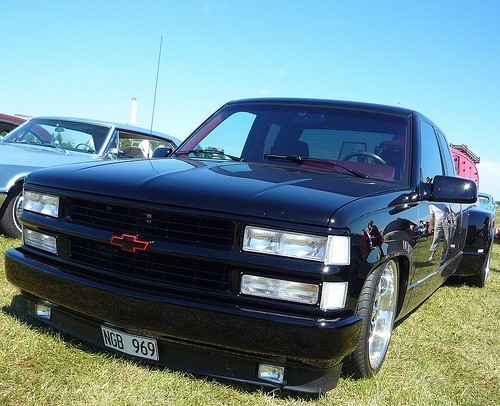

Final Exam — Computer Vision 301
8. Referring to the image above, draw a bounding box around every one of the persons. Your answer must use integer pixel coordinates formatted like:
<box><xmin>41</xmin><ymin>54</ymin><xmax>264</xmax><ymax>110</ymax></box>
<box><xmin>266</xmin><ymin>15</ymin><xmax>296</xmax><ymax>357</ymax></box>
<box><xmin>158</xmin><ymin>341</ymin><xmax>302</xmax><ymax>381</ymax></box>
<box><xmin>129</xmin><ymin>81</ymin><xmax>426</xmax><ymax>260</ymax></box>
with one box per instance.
<box><xmin>362</xmin><ymin>220</ymin><xmax>384</xmax><ymax>248</ymax></box>
<box><xmin>383</xmin><ymin>218</ymin><xmax>417</xmax><ymax>242</ymax></box>
<box><xmin>418</xmin><ymin>220</ymin><xmax>429</xmax><ymax>241</ymax></box>
<box><xmin>424</xmin><ymin>203</ymin><xmax>456</xmax><ymax>263</ymax></box>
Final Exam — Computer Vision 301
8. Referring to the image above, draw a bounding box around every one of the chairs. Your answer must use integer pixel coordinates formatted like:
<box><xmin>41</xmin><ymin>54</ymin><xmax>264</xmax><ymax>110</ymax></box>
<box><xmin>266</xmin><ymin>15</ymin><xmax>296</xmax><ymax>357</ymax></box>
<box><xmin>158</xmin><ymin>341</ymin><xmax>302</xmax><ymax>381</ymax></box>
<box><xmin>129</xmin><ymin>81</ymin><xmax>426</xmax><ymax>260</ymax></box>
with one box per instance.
<box><xmin>123</xmin><ymin>147</ymin><xmax>144</xmax><ymax>159</ymax></box>
<box><xmin>373</xmin><ymin>141</ymin><xmax>403</xmax><ymax>180</ymax></box>
<box><xmin>270</xmin><ymin>121</ymin><xmax>310</xmax><ymax>160</ymax></box>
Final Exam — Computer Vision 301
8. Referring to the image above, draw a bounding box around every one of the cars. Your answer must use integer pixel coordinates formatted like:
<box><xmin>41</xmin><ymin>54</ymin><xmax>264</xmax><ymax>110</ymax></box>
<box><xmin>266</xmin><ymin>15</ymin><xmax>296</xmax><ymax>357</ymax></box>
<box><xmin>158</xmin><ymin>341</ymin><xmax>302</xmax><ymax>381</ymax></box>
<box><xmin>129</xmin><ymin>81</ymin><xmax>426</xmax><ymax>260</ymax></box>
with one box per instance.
<box><xmin>0</xmin><ymin>116</ymin><xmax>197</xmax><ymax>240</ymax></box>
<box><xmin>4</xmin><ymin>97</ymin><xmax>496</xmax><ymax>394</ymax></box>
<box><xmin>0</xmin><ymin>113</ymin><xmax>55</xmax><ymax>144</ymax></box>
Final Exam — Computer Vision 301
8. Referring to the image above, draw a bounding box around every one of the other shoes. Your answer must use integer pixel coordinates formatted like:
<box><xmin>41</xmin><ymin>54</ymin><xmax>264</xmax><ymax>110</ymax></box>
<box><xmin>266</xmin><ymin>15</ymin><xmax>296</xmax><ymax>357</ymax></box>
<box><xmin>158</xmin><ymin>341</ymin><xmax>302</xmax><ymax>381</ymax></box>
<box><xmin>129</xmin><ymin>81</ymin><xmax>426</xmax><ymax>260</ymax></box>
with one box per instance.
<box><xmin>426</xmin><ymin>257</ymin><xmax>434</xmax><ymax>263</ymax></box>
<box><xmin>440</xmin><ymin>254</ymin><xmax>445</xmax><ymax>260</ymax></box>
